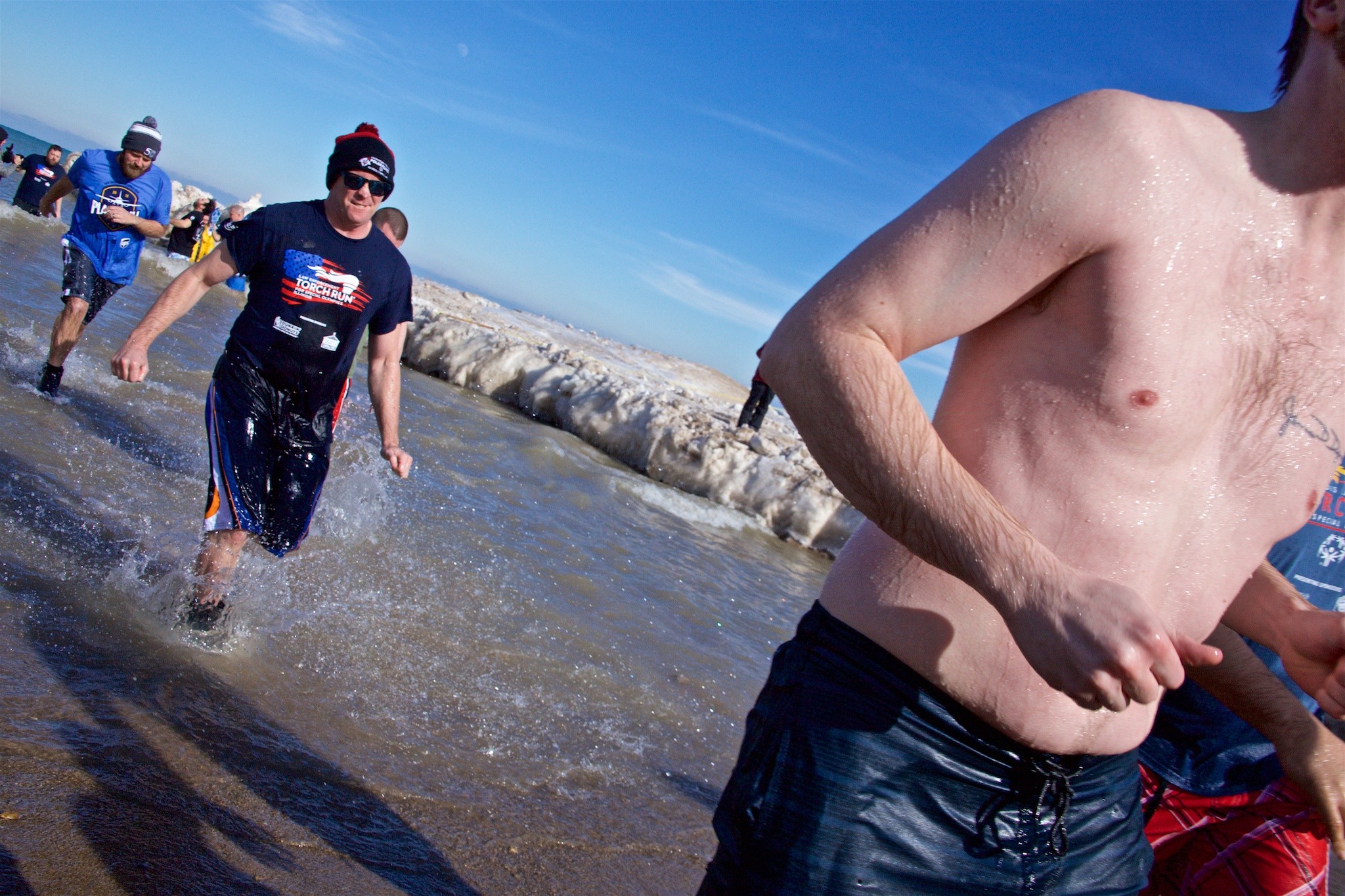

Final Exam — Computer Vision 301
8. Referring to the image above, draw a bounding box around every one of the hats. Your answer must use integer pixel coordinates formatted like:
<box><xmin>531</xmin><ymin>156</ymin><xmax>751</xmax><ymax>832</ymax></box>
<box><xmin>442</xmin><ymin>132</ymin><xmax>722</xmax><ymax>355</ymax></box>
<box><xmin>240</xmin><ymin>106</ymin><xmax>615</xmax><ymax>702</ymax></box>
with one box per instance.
<box><xmin>121</xmin><ymin>116</ymin><xmax>161</xmax><ymax>160</ymax></box>
<box><xmin>326</xmin><ymin>123</ymin><xmax>396</xmax><ymax>203</ymax></box>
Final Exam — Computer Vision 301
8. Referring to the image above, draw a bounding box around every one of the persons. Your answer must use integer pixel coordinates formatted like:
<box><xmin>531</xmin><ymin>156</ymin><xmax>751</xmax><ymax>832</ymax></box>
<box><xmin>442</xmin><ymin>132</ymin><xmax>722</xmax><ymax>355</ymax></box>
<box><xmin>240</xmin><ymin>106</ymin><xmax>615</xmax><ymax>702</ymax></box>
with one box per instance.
<box><xmin>111</xmin><ymin>121</ymin><xmax>413</xmax><ymax>573</ymax></box>
<box><xmin>38</xmin><ymin>116</ymin><xmax>172</xmax><ymax>397</ymax></box>
<box><xmin>737</xmin><ymin>342</ymin><xmax>776</xmax><ymax>433</ymax></box>
<box><xmin>0</xmin><ymin>127</ymin><xmax>22</xmax><ymax>179</ymax></box>
<box><xmin>332</xmin><ymin>207</ymin><xmax>409</xmax><ymax>434</ymax></box>
<box><xmin>12</xmin><ymin>145</ymin><xmax>66</xmax><ymax>217</ymax></box>
<box><xmin>694</xmin><ymin>1</ymin><xmax>1345</xmax><ymax>896</ymax></box>
<box><xmin>169</xmin><ymin>197</ymin><xmax>246</xmax><ymax>292</ymax></box>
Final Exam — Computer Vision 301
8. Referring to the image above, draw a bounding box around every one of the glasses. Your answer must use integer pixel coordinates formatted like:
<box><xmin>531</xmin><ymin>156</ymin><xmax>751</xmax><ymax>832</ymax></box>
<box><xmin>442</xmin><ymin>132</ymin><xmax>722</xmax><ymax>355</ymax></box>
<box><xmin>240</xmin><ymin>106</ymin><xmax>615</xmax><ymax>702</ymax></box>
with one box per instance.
<box><xmin>341</xmin><ymin>171</ymin><xmax>392</xmax><ymax>196</ymax></box>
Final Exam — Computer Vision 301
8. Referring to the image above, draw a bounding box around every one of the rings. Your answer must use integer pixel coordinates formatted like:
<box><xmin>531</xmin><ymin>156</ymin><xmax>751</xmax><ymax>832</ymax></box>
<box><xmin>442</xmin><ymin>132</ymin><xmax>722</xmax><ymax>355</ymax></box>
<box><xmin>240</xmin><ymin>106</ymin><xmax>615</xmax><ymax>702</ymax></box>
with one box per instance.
<box><xmin>113</xmin><ymin>213</ymin><xmax>114</xmax><ymax>218</ymax></box>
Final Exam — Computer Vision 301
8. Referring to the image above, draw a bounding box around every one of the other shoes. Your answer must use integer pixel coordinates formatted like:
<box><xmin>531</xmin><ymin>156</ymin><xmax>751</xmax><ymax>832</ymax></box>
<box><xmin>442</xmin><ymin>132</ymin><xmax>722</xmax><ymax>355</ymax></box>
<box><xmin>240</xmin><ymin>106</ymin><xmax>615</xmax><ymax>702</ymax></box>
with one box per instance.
<box><xmin>38</xmin><ymin>361</ymin><xmax>64</xmax><ymax>397</ymax></box>
<box><xmin>188</xmin><ymin>596</ymin><xmax>227</xmax><ymax>633</ymax></box>
